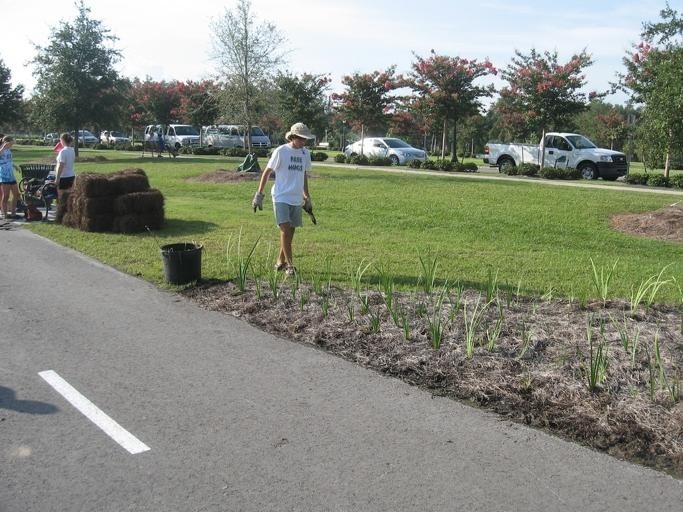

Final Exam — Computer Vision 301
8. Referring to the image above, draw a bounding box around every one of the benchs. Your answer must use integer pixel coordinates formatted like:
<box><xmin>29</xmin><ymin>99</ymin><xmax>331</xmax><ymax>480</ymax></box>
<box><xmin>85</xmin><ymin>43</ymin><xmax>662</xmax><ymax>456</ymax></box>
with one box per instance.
<box><xmin>19</xmin><ymin>171</ymin><xmax>57</xmax><ymax>221</ymax></box>
<box><xmin>143</xmin><ymin>140</ymin><xmax>176</xmax><ymax>158</ymax></box>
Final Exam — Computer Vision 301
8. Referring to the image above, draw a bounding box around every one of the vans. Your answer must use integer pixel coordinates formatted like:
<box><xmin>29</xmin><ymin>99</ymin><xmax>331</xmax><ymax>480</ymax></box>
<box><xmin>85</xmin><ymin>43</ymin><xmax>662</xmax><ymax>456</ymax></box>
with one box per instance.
<box><xmin>144</xmin><ymin>124</ymin><xmax>271</xmax><ymax>151</ymax></box>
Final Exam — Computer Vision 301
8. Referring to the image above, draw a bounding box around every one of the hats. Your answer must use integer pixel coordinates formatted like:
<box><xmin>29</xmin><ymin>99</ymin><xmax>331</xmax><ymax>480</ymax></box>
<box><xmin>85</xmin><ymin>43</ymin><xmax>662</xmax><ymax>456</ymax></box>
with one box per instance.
<box><xmin>282</xmin><ymin>122</ymin><xmax>316</xmax><ymax>141</ymax></box>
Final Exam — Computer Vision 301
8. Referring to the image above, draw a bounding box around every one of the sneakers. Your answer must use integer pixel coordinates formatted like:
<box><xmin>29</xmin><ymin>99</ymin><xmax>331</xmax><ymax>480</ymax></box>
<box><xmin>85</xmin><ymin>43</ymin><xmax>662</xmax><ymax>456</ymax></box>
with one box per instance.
<box><xmin>286</xmin><ymin>264</ymin><xmax>296</xmax><ymax>275</ymax></box>
<box><xmin>3</xmin><ymin>214</ymin><xmax>22</xmax><ymax>219</ymax></box>
<box><xmin>274</xmin><ymin>261</ymin><xmax>285</xmax><ymax>272</ymax></box>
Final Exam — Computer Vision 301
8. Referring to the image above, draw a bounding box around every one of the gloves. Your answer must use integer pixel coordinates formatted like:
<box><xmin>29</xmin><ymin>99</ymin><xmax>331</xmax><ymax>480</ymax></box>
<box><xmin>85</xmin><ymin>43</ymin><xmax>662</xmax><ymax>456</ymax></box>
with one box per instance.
<box><xmin>303</xmin><ymin>198</ymin><xmax>313</xmax><ymax>214</ymax></box>
<box><xmin>251</xmin><ymin>189</ymin><xmax>265</xmax><ymax>214</ymax></box>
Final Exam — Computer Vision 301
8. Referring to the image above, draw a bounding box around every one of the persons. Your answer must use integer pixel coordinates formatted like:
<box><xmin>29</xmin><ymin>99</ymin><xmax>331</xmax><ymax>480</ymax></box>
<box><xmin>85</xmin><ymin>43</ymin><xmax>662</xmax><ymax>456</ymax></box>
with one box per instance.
<box><xmin>0</xmin><ymin>135</ymin><xmax>21</xmax><ymax>219</ymax></box>
<box><xmin>54</xmin><ymin>133</ymin><xmax>75</xmax><ymax>198</ymax></box>
<box><xmin>148</xmin><ymin>133</ymin><xmax>156</xmax><ymax>158</ymax></box>
<box><xmin>156</xmin><ymin>133</ymin><xmax>165</xmax><ymax>158</ymax></box>
<box><xmin>251</xmin><ymin>122</ymin><xmax>317</xmax><ymax>274</ymax></box>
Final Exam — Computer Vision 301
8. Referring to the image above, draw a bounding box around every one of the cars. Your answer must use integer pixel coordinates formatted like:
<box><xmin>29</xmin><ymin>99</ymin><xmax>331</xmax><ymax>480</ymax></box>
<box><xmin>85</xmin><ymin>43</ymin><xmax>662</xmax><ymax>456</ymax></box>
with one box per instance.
<box><xmin>44</xmin><ymin>133</ymin><xmax>59</xmax><ymax>143</ymax></box>
<box><xmin>70</xmin><ymin>130</ymin><xmax>131</xmax><ymax>144</ymax></box>
<box><xmin>345</xmin><ymin>137</ymin><xmax>428</xmax><ymax>165</ymax></box>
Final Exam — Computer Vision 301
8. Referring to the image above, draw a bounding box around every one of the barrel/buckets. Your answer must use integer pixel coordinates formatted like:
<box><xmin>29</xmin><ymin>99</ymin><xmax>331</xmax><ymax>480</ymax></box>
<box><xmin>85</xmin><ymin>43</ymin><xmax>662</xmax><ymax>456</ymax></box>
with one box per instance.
<box><xmin>161</xmin><ymin>242</ymin><xmax>202</xmax><ymax>285</ymax></box>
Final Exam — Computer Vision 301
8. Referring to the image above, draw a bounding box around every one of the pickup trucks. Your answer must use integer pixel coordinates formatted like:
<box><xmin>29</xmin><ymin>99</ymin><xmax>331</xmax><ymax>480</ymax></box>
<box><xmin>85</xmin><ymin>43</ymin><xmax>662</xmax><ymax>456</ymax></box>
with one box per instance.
<box><xmin>482</xmin><ymin>132</ymin><xmax>627</xmax><ymax>181</ymax></box>
